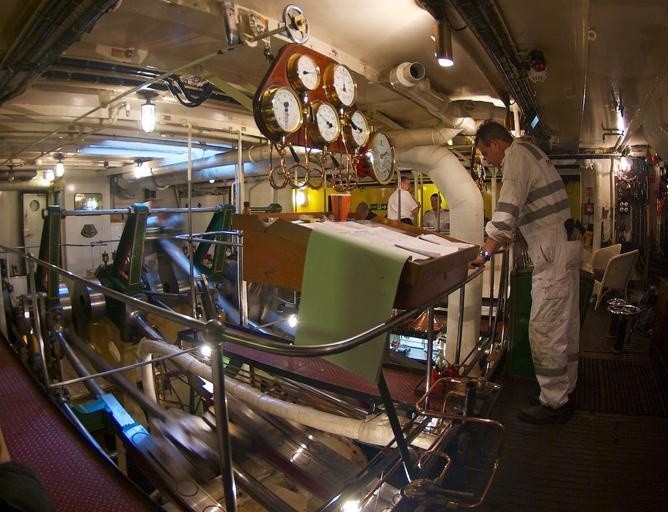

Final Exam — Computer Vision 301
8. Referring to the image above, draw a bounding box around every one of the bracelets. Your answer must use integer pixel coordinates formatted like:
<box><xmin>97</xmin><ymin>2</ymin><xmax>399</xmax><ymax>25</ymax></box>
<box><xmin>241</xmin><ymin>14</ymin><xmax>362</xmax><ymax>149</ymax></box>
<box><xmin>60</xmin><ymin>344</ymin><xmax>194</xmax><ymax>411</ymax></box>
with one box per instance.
<box><xmin>479</xmin><ymin>247</ymin><xmax>490</xmax><ymax>261</ymax></box>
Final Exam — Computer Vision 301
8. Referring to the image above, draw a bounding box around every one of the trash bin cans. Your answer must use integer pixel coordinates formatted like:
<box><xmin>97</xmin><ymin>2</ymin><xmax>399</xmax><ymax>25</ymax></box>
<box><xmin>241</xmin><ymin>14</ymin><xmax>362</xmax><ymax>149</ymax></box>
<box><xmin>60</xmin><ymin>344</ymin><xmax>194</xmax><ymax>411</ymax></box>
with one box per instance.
<box><xmin>510</xmin><ymin>268</ymin><xmax>594</xmax><ymax>378</ymax></box>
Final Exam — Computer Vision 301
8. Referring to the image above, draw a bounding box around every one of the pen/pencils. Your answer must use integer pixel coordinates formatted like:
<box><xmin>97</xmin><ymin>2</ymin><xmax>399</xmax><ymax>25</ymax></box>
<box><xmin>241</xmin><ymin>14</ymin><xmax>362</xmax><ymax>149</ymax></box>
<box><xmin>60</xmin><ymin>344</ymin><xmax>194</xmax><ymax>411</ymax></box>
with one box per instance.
<box><xmin>418</xmin><ymin>237</ymin><xmax>441</xmax><ymax>245</ymax></box>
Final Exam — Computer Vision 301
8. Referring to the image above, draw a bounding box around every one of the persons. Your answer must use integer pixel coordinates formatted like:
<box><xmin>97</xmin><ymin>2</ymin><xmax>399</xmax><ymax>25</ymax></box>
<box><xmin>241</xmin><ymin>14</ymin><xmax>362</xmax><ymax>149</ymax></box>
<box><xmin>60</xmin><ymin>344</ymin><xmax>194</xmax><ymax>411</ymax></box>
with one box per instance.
<box><xmin>467</xmin><ymin>118</ymin><xmax>584</xmax><ymax>425</ymax></box>
<box><xmin>357</xmin><ymin>201</ymin><xmax>377</xmax><ymax>220</ymax></box>
<box><xmin>386</xmin><ymin>175</ymin><xmax>419</xmax><ymax>226</ymax></box>
<box><xmin>423</xmin><ymin>193</ymin><xmax>450</xmax><ymax>231</ymax></box>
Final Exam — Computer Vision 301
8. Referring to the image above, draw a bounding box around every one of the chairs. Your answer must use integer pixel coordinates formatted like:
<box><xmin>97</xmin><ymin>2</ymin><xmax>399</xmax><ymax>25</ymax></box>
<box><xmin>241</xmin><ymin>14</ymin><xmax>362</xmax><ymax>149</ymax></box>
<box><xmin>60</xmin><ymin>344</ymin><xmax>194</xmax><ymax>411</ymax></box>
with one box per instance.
<box><xmin>592</xmin><ymin>248</ymin><xmax>639</xmax><ymax>311</ymax></box>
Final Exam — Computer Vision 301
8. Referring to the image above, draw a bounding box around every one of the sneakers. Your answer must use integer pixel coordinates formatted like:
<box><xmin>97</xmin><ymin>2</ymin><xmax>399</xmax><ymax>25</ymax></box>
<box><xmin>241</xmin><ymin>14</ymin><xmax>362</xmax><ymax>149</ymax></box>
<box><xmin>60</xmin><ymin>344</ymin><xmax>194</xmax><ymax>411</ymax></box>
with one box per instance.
<box><xmin>530</xmin><ymin>391</ymin><xmax>575</xmax><ymax>406</ymax></box>
<box><xmin>518</xmin><ymin>403</ymin><xmax>571</xmax><ymax>426</ymax></box>
